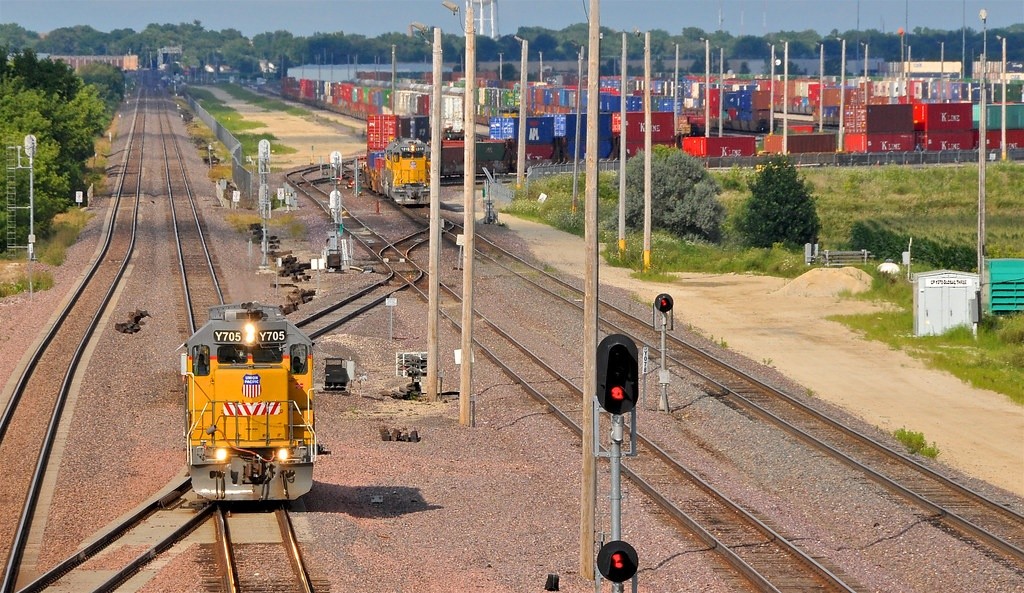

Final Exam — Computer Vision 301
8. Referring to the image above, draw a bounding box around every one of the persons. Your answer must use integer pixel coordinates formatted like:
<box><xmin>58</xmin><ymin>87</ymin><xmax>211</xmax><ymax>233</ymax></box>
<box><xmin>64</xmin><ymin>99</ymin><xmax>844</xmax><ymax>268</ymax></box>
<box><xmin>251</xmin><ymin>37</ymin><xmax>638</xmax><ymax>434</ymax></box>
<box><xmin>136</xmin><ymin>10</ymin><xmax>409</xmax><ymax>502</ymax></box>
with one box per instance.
<box><xmin>198</xmin><ymin>354</ymin><xmax>207</xmax><ymax>374</ymax></box>
<box><xmin>291</xmin><ymin>356</ymin><xmax>303</xmax><ymax>373</ymax></box>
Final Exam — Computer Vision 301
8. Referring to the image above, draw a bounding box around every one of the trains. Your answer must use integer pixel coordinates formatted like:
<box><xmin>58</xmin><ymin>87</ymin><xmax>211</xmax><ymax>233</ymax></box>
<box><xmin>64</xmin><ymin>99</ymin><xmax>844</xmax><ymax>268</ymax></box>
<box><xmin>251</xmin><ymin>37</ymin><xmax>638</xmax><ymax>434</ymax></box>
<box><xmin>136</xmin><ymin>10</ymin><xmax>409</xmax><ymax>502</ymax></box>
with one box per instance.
<box><xmin>170</xmin><ymin>301</ymin><xmax>325</xmax><ymax>504</ymax></box>
<box><xmin>363</xmin><ymin>109</ymin><xmax>676</xmax><ymax>208</ymax></box>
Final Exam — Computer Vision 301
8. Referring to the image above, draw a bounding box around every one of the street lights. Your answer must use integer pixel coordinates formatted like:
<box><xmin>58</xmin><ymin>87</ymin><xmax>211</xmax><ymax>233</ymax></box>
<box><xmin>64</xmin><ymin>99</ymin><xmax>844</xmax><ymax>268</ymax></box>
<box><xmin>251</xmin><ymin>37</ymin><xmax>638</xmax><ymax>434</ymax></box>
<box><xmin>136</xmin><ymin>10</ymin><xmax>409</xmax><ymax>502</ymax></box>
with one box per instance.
<box><xmin>979</xmin><ymin>6</ymin><xmax>988</xmax><ymax>290</ymax></box>
<box><xmin>513</xmin><ymin>36</ymin><xmax>529</xmax><ymax>190</ymax></box>
<box><xmin>836</xmin><ymin>36</ymin><xmax>846</xmax><ymax>153</ymax></box>
<box><xmin>780</xmin><ymin>39</ymin><xmax>787</xmax><ymax>157</ymax></box>
<box><xmin>995</xmin><ymin>34</ymin><xmax>1007</xmax><ymax>162</ymax></box>
<box><xmin>699</xmin><ymin>36</ymin><xmax>710</xmax><ymax>138</ymax></box>
<box><xmin>671</xmin><ymin>41</ymin><xmax>679</xmax><ymax>136</ymax></box>
<box><xmin>406</xmin><ymin>21</ymin><xmax>442</xmax><ymax>401</ymax></box>
<box><xmin>442</xmin><ymin>0</ymin><xmax>477</xmax><ymax>432</ymax></box>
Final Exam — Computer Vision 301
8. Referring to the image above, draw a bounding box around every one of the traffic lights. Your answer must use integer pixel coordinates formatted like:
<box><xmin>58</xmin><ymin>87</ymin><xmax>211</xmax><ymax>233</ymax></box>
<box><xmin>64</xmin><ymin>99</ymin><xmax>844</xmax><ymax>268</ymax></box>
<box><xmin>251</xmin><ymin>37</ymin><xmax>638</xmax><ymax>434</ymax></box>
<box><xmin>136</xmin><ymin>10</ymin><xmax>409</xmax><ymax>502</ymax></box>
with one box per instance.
<box><xmin>597</xmin><ymin>540</ymin><xmax>639</xmax><ymax>583</ymax></box>
<box><xmin>596</xmin><ymin>334</ymin><xmax>639</xmax><ymax>415</ymax></box>
<box><xmin>654</xmin><ymin>293</ymin><xmax>673</xmax><ymax>313</ymax></box>
